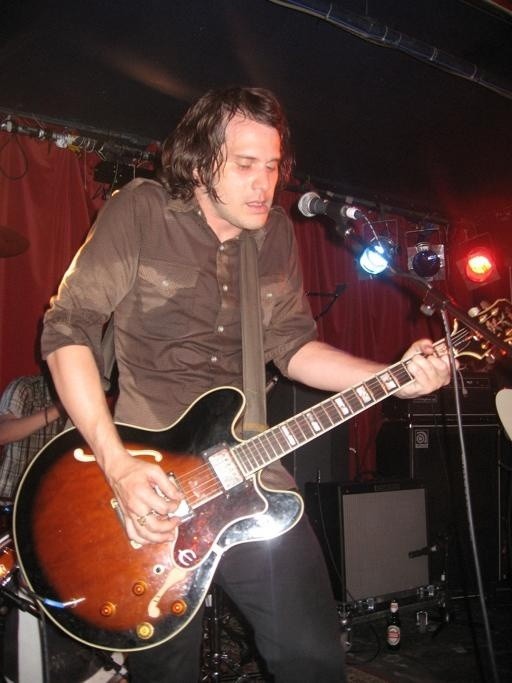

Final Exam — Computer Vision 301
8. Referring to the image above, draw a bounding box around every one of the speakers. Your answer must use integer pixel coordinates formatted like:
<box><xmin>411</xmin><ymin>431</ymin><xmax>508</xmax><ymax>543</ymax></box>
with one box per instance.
<box><xmin>304</xmin><ymin>476</ymin><xmax>432</xmax><ymax>607</ymax></box>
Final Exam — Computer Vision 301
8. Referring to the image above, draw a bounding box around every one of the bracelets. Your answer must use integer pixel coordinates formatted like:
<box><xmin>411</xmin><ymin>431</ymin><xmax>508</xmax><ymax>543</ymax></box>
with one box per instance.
<box><xmin>44</xmin><ymin>405</ymin><xmax>53</xmax><ymax>428</ymax></box>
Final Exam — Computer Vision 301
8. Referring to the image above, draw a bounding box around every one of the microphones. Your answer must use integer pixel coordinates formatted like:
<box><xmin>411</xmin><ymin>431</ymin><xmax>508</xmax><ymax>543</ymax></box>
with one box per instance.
<box><xmin>297</xmin><ymin>191</ymin><xmax>365</xmax><ymax>223</ymax></box>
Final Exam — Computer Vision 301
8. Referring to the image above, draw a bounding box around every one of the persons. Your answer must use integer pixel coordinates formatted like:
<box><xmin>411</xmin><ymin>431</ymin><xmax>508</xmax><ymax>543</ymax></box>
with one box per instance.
<box><xmin>38</xmin><ymin>75</ymin><xmax>460</xmax><ymax>682</ymax></box>
<box><xmin>1</xmin><ymin>318</ymin><xmax>86</xmax><ymax>516</ymax></box>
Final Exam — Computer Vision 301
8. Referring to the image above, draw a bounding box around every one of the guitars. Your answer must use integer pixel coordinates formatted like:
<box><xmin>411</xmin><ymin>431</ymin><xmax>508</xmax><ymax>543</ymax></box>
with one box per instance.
<box><xmin>12</xmin><ymin>298</ymin><xmax>511</xmax><ymax>652</ymax></box>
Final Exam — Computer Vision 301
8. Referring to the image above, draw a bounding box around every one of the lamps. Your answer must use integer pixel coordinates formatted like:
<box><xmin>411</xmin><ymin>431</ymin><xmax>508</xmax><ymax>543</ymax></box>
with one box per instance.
<box><xmin>356</xmin><ymin>234</ymin><xmax>502</xmax><ymax>292</ymax></box>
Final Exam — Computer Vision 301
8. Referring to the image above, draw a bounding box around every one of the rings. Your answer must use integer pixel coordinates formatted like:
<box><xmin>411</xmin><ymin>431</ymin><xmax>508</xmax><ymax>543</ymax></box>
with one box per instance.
<box><xmin>136</xmin><ymin>511</ymin><xmax>154</xmax><ymax>527</ymax></box>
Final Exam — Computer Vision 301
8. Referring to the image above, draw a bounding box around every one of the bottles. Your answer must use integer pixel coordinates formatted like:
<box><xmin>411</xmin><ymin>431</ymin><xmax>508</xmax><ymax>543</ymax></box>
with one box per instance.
<box><xmin>385</xmin><ymin>598</ymin><xmax>404</xmax><ymax>651</ymax></box>
